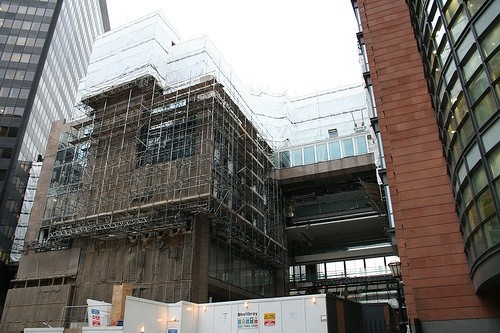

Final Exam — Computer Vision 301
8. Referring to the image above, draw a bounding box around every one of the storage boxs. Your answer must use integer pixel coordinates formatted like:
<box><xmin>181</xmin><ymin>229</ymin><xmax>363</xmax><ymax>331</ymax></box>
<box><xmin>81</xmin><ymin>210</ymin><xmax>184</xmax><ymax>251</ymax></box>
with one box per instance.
<box><xmin>111</xmin><ymin>283</ymin><xmax>132</xmax><ymax>325</ymax></box>
<box><xmin>86</xmin><ymin>298</ymin><xmax>113</xmax><ymax>326</ymax></box>
